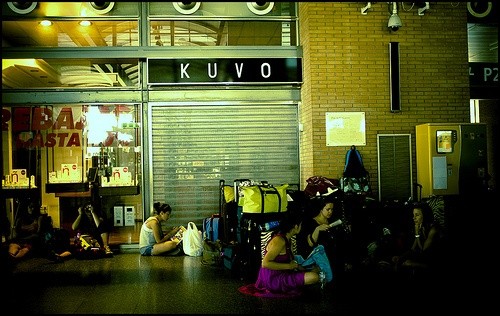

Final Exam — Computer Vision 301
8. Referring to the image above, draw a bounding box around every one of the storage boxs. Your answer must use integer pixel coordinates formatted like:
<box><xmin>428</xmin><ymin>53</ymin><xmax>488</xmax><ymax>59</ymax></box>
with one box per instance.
<box><xmin>202</xmin><ymin>178</ymin><xmax>434</xmax><ymax>278</ymax></box>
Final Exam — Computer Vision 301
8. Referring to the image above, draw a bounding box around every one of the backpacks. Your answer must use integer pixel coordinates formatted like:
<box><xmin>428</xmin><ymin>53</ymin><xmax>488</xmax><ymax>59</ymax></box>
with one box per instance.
<box><xmin>202</xmin><ymin>238</ymin><xmax>222</xmax><ymax>266</ymax></box>
<box><xmin>224</xmin><ymin>242</ymin><xmax>241</xmax><ymax>270</ymax></box>
<box><xmin>340</xmin><ymin>145</ymin><xmax>372</xmax><ymax>195</ymax></box>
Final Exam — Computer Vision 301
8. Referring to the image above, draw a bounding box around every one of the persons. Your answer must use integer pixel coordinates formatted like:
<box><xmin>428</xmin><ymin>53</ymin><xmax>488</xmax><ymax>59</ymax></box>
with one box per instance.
<box><xmin>401</xmin><ymin>203</ymin><xmax>443</xmax><ymax>278</ymax></box>
<box><xmin>238</xmin><ymin>214</ymin><xmax>320</xmax><ymax>300</ymax></box>
<box><xmin>70</xmin><ymin>201</ymin><xmax>114</xmax><ymax>255</ymax></box>
<box><xmin>295</xmin><ymin>199</ymin><xmax>352</xmax><ymax>290</ymax></box>
<box><xmin>138</xmin><ymin>202</ymin><xmax>181</xmax><ymax>257</ymax></box>
<box><xmin>7</xmin><ymin>198</ymin><xmax>43</xmax><ymax>264</ymax></box>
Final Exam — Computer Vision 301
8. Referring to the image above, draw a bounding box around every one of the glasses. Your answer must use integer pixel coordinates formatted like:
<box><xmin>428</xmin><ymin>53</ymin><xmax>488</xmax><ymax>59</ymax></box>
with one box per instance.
<box><xmin>164</xmin><ymin>204</ymin><xmax>169</xmax><ymax>210</ymax></box>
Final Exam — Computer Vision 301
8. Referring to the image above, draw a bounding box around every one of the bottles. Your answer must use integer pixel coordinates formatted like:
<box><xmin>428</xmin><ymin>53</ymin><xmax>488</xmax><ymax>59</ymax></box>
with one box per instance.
<box><xmin>258</xmin><ymin>220</ymin><xmax>279</xmax><ymax>230</ymax></box>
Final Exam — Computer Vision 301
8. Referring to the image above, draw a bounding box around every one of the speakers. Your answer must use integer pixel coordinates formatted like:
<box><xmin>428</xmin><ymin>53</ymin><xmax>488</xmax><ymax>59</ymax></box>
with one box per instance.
<box><xmin>388</xmin><ymin>42</ymin><xmax>401</xmax><ymax>113</ymax></box>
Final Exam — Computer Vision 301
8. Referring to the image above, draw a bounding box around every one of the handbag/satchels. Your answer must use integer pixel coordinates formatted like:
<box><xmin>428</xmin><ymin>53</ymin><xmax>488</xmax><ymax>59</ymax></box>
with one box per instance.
<box><xmin>291</xmin><ymin>245</ymin><xmax>333</xmax><ymax>289</ymax></box>
<box><xmin>306</xmin><ymin>176</ymin><xmax>339</xmax><ymax>199</ymax></box>
<box><xmin>182</xmin><ymin>222</ymin><xmax>204</xmax><ymax>257</ymax></box>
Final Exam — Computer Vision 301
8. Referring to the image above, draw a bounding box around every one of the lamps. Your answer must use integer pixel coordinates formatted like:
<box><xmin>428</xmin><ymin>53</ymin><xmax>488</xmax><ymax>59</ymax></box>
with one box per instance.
<box><xmin>77</xmin><ymin>2</ymin><xmax>94</xmax><ymax>27</ymax></box>
<box><xmin>38</xmin><ymin>4</ymin><xmax>54</xmax><ymax>29</ymax></box>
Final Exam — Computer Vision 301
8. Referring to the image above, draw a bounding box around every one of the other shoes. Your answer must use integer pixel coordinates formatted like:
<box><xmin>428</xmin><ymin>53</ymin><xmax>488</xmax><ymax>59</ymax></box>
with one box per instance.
<box><xmin>103</xmin><ymin>247</ymin><xmax>113</xmax><ymax>255</ymax></box>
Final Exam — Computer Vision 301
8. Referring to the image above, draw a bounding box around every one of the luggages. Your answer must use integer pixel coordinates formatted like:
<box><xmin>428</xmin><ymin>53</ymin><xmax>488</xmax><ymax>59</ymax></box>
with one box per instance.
<box><xmin>223</xmin><ymin>180</ymin><xmax>289</xmax><ymax>213</ymax></box>
<box><xmin>203</xmin><ymin>214</ymin><xmax>224</xmax><ymax>241</ymax></box>
